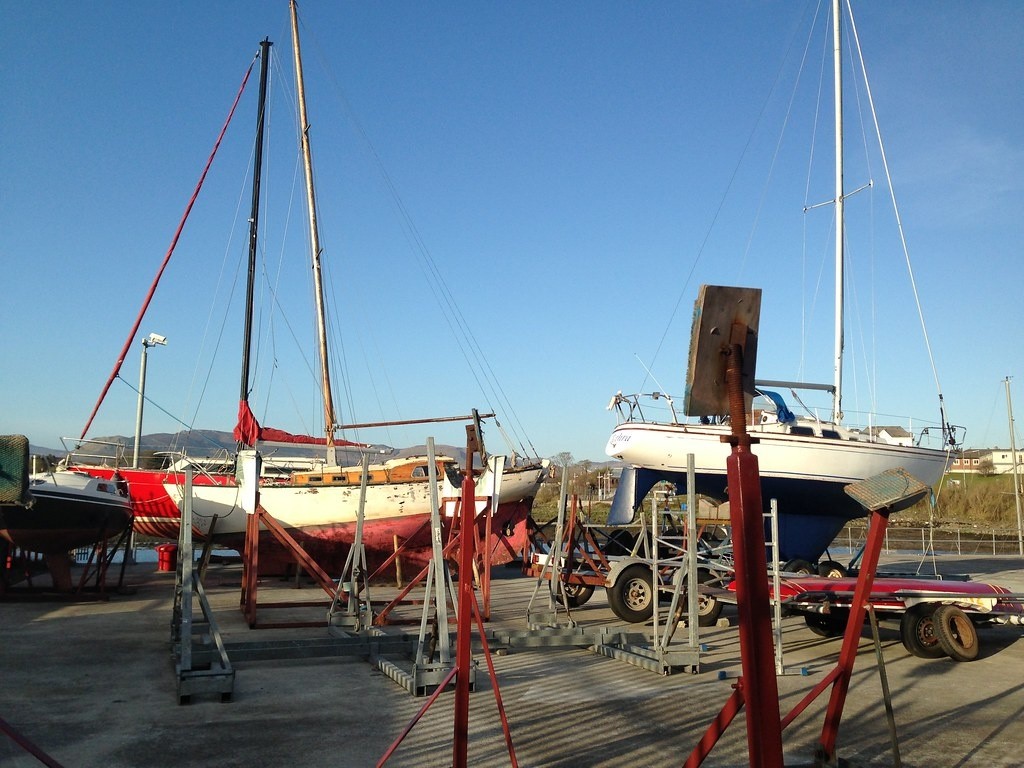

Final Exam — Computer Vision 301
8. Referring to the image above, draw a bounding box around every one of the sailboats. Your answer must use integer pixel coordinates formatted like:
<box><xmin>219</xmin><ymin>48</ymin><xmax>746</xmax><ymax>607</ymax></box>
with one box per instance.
<box><xmin>608</xmin><ymin>0</ymin><xmax>967</xmax><ymax>565</ymax></box>
<box><xmin>57</xmin><ymin>35</ymin><xmax>553</xmax><ymax>577</ymax></box>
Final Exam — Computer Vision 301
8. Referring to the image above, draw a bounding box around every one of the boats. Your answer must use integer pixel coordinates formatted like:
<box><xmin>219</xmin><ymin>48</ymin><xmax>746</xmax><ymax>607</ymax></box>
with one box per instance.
<box><xmin>0</xmin><ymin>470</ymin><xmax>135</xmax><ymax>592</ymax></box>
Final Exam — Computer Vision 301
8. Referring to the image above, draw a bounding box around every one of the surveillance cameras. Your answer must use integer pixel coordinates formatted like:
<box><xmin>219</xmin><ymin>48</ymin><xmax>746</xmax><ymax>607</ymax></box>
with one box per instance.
<box><xmin>607</xmin><ymin>396</ymin><xmax>617</xmax><ymax>411</ymax></box>
<box><xmin>149</xmin><ymin>333</ymin><xmax>168</xmax><ymax>345</ymax></box>
